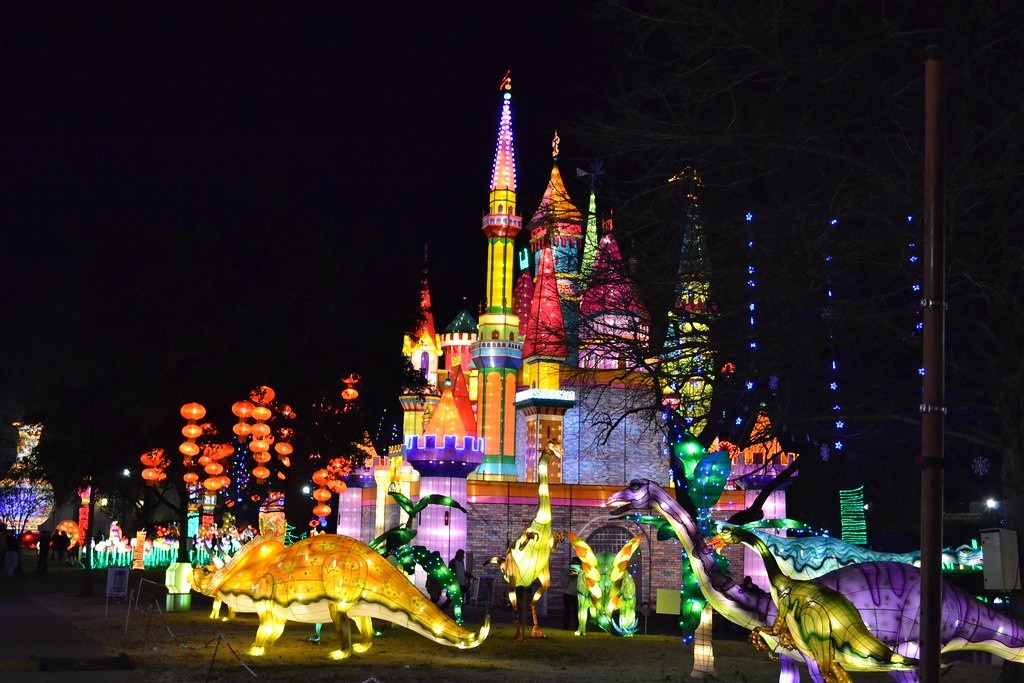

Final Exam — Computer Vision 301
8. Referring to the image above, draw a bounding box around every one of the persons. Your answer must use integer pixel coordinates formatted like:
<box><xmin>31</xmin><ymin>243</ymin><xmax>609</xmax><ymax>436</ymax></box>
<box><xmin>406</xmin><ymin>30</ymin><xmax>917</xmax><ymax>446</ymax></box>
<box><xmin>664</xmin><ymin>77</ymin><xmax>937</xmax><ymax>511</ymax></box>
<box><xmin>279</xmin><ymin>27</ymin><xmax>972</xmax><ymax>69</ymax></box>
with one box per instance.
<box><xmin>5</xmin><ymin>527</ymin><xmax>85</xmax><ymax>579</ymax></box>
<box><xmin>191</xmin><ymin>533</ymin><xmax>256</xmax><ymax>558</ymax></box>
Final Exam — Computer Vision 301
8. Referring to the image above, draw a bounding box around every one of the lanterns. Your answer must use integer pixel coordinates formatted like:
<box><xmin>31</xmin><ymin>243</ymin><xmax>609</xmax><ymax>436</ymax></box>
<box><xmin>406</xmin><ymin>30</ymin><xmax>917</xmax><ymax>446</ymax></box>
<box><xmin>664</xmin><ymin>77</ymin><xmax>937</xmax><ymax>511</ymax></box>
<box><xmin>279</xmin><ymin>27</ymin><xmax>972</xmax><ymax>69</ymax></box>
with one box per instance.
<box><xmin>141</xmin><ymin>373</ymin><xmax>362</xmax><ymax>517</ymax></box>
